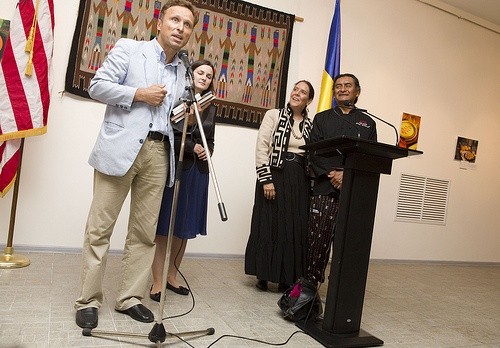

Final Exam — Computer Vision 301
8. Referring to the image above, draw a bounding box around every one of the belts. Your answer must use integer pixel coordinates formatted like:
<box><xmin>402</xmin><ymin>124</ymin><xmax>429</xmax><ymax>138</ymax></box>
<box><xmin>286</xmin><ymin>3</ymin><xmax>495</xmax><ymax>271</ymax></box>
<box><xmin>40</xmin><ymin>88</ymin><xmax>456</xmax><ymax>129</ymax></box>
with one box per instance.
<box><xmin>147</xmin><ymin>131</ymin><xmax>169</xmax><ymax>142</ymax></box>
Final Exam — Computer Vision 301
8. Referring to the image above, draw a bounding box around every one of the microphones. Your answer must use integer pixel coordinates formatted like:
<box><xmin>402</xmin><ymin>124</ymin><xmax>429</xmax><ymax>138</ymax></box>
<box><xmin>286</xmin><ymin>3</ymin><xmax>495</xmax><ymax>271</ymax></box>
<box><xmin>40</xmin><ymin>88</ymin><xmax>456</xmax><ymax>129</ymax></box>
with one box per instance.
<box><xmin>344</xmin><ymin>100</ymin><xmax>399</xmax><ymax>146</ymax></box>
<box><xmin>178</xmin><ymin>49</ymin><xmax>194</xmax><ymax>80</ymax></box>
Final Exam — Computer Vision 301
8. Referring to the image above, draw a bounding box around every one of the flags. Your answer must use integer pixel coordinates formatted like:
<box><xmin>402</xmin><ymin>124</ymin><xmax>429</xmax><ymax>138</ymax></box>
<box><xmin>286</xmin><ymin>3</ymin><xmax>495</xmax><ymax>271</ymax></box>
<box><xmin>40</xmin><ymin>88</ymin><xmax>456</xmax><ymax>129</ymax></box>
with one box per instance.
<box><xmin>0</xmin><ymin>0</ymin><xmax>55</xmax><ymax>198</ymax></box>
<box><xmin>316</xmin><ymin>0</ymin><xmax>342</xmax><ymax>113</ymax></box>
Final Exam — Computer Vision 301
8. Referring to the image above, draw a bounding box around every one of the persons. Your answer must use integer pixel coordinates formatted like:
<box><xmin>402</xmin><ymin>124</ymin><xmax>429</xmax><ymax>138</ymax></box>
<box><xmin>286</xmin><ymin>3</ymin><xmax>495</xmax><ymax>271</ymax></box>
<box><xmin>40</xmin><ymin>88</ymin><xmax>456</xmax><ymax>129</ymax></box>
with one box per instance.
<box><xmin>244</xmin><ymin>80</ymin><xmax>314</xmax><ymax>293</ymax></box>
<box><xmin>307</xmin><ymin>73</ymin><xmax>377</xmax><ymax>287</ymax></box>
<box><xmin>73</xmin><ymin>0</ymin><xmax>198</xmax><ymax>329</ymax></box>
<box><xmin>148</xmin><ymin>60</ymin><xmax>215</xmax><ymax>303</ymax></box>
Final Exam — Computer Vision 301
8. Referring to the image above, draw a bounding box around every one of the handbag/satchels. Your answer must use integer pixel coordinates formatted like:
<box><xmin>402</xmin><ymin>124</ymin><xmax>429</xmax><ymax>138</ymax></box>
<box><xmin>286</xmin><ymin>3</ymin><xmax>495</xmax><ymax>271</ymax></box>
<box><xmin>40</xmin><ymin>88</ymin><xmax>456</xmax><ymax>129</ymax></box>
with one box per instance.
<box><xmin>277</xmin><ymin>276</ymin><xmax>323</xmax><ymax>322</ymax></box>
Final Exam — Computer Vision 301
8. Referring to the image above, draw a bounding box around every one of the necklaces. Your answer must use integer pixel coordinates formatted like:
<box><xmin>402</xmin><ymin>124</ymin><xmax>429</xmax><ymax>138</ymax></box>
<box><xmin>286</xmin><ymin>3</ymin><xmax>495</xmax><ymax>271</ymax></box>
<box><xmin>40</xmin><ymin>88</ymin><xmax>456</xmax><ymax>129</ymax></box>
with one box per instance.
<box><xmin>285</xmin><ymin>108</ymin><xmax>305</xmax><ymax>139</ymax></box>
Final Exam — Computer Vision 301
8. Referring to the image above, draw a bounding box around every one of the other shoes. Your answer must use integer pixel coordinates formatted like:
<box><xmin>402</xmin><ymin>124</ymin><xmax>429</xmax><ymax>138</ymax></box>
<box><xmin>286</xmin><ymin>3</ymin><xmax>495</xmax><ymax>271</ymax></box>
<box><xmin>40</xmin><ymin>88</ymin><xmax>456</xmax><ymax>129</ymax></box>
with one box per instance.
<box><xmin>167</xmin><ymin>281</ymin><xmax>189</xmax><ymax>296</ymax></box>
<box><xmin>150</xmin><ymin>283</ymin><xmax>161</xmax><ymax>302</ymax></box>
<box><xmin>279</xmin><ymin>284</ymin><xmax>289</xmax><ymax>293</ymax></box>
<box><xmin>255</xmin><ymin>279</ymin><xmax>269</xmax><ymax>291</ymax></box>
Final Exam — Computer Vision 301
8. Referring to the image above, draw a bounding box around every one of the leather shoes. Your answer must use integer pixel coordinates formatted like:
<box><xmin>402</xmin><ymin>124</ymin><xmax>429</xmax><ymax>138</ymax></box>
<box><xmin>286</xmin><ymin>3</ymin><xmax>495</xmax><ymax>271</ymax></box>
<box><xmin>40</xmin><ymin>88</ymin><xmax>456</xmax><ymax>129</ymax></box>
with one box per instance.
<box><xmin>115</xmin><ymin>304</ymin><xmax>155</xmax><ymax>323</ymax></box>
<box><xmin>75</xmin><ymin>307</ymin><xmax>98</xmax><ymax>329</ymax></box>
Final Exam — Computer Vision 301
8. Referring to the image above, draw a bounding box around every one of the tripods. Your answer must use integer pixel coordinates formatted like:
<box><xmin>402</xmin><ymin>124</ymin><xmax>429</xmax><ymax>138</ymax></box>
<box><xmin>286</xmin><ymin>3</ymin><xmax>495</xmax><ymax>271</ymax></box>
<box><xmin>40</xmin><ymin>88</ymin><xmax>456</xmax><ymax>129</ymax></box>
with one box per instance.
<box><xmin>82</xmin><ymin>71</ymin><xmax>227</xmax><ymax>347</ymax></box>
